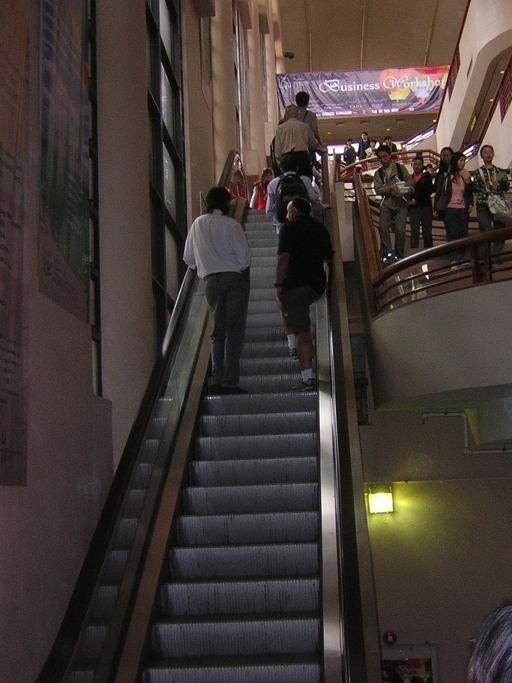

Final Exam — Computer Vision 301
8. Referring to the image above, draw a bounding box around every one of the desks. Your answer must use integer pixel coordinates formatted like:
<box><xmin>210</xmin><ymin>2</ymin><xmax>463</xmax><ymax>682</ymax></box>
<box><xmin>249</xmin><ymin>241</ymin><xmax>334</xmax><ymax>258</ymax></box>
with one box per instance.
<box><xmin>380</xmin><ymin>643</ymin><xmax>440</xmax><ymax>682</ymax></box>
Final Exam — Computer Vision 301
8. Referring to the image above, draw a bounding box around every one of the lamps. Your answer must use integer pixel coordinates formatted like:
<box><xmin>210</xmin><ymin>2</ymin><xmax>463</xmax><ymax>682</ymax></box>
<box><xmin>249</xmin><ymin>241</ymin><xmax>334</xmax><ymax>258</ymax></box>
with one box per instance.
<box><xmin>366</xmin><ymin>483</ymin><xmax>395</xmax><ymax>515</ymax></box>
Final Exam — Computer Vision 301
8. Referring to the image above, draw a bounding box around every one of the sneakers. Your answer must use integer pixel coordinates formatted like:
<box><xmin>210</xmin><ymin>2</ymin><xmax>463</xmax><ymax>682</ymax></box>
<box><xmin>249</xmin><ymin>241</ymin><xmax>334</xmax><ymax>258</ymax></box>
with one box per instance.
<box><xmin>287</xmin><ymin>377</ymin><xmax>316</xmax><ymax>392</ymax></box>
<box><xmin>447</xmin><ymin>259</ymin><xmax>471</xmax><ymax>273</ymax></box>
<box><xmin>286</xmin><ymin>347</ymin><xmax>298</xmax><ymax>360</ymax></box>
<box><xmin>208</xmin><ymin>384</ymin><xmax>250</xmax><ymax>395</ymax></box>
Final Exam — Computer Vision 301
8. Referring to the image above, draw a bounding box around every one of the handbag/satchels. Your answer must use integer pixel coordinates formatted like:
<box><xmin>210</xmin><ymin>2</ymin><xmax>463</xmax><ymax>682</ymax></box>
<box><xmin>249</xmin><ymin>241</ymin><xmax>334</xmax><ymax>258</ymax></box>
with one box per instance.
<box><xmin>486</xmin><ymin>192</ymin><xmax>512</xmax><ymax>224</ymax></box>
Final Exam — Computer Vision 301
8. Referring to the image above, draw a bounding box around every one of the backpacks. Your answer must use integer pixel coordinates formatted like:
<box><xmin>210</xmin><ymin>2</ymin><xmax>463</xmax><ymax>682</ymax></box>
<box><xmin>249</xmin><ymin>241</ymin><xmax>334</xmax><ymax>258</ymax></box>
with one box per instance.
<box><xmin>274</xmin><ymin>171</ymin><xmax>312</xmax><ymax>223</ymax></box>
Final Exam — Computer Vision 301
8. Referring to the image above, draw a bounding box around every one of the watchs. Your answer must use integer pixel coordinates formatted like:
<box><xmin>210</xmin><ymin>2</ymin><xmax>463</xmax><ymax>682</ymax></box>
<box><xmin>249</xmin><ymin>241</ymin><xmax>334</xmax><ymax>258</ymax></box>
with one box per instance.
<box><xmin>274</xmin><ymin>283</ymin><xmax>282</xmax><ymax>288</ymax></box>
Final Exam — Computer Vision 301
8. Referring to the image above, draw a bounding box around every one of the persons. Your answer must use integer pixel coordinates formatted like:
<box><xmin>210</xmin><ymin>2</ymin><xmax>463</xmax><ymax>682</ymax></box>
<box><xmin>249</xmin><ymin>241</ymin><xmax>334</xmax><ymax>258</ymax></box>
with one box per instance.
<box><xmin>181</xmin><ymin>186</ymin><xmax>252</xmax><ymax>394</ymax></box>
<box><xmin>273</xmin><ymin>196</ymin><xmax>334</xmax><ymax>391</ymax></box>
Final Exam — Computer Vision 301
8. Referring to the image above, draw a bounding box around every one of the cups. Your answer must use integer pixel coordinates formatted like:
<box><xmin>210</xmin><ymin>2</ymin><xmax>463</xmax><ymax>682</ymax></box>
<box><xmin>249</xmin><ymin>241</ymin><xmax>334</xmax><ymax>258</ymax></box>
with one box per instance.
<box><xmin>396</xmin><ymin>181</ymin><xmax>405</xmax><ymax>190</ymax></box>
